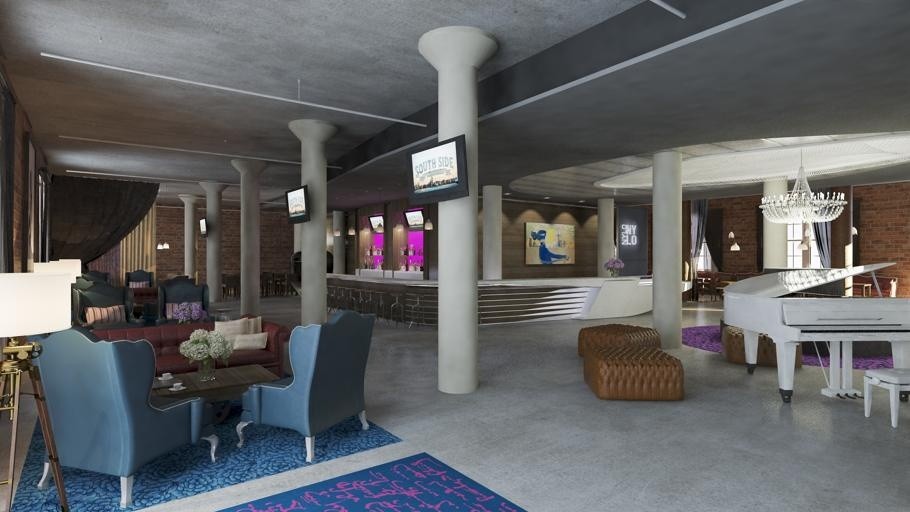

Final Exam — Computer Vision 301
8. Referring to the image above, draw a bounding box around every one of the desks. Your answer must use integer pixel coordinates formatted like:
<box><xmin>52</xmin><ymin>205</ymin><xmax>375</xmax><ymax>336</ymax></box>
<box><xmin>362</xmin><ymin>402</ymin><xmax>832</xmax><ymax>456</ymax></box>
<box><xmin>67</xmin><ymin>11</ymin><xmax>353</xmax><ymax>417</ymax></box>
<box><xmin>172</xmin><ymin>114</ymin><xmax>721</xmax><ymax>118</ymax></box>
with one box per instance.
<box><xmin>216</xmin><ymin>307</ymin><xmax>234</xmax><ymax>320</ymax></box>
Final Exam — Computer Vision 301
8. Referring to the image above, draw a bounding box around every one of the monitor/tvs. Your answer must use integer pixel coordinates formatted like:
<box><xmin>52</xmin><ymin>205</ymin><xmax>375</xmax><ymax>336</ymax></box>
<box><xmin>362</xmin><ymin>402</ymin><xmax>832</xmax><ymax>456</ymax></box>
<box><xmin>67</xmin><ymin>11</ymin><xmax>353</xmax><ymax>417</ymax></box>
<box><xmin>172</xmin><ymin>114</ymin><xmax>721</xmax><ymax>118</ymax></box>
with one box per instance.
<box><xmin>407</xmin><ymin>134</ymin><xmax>469</xmax><ymax>206</ymax></box>
<box><xmin>199</xmin><ymin>218</ymin><xmax>208</xmax><ymax>238</ymax></box>
<box><xmin>285</xmin><ymin>185</ymin><xmax>311</xmax><ymax>224</ymax></box>
<box><xmin>367</xmin><ymin>215</ymin><xmax>384</xmax><ymax>234</ymax></box>
<box><xmin>403</xmin><ymin>210</ymin><xmax>425</xmax><ymax>227</ymax></box>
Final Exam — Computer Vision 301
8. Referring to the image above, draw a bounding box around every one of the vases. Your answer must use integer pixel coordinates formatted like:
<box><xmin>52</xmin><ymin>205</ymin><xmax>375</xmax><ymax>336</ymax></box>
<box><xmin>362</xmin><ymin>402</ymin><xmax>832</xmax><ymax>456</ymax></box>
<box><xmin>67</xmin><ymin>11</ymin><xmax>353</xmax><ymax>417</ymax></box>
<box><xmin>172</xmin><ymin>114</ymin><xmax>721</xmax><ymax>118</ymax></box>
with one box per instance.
<box><xmin>199</xmin><ymin>357</ymin><xmax>217</xmax><ymax>382</ymax></box>
<box><xmin>610</xmin><ymin>269</ymin><xmax>622</xmax><ymax>278</ymax></box>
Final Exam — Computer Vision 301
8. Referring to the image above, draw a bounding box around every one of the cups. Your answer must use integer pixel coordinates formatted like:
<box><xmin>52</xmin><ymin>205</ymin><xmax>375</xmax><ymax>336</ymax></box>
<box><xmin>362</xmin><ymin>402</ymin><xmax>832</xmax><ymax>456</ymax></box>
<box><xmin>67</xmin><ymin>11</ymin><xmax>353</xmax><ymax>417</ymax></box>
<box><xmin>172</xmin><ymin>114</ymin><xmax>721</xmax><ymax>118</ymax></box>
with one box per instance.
<box><xmin>173</xmin><ymin>383</ymin><xmax>183</xmax><ymax>389</ymax></box>
<box><xmin>162</xmin><ymin>373</ymin><xmax>171</xmax><ymax>378</ymax></box>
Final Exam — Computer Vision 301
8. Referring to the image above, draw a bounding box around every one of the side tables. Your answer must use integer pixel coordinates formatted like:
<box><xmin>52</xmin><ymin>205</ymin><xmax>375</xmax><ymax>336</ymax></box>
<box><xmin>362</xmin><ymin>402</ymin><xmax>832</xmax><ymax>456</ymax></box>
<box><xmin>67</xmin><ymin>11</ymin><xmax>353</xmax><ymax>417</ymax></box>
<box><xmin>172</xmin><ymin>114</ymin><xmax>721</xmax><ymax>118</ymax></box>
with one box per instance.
<box><xmin>853</xmin><ymin>281</ymin><xmax>873</xmax><ymax>298</ymax></box>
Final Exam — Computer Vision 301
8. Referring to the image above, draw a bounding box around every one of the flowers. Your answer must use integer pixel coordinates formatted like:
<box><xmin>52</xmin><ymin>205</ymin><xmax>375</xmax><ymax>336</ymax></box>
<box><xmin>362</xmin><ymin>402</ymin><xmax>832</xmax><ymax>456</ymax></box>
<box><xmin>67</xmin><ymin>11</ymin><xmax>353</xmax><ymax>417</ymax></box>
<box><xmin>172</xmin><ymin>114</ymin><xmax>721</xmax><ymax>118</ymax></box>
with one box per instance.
<box><xmin>179</xmin><ymin>328</ymin><xmax>233</xmax><ymax>359</ymax></box>
<box><xmin>604</xmin><ymin>257</ymin><xmax>625</xmax><ymax>273</ymax></box>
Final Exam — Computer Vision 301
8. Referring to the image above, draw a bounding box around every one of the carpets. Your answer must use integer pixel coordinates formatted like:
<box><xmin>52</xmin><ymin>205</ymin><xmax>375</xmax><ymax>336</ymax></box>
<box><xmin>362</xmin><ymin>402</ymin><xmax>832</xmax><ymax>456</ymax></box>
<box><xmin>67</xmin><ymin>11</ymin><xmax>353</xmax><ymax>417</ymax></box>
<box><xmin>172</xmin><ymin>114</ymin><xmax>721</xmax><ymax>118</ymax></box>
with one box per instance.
<box><xmin>10</xmin><ymin>372</ymin><xmax>403</xmax><ymax>511</ymax></box>
<box><xmin>682</xmin><ymin>324</ymin><xmax>894</xmax><ymax>370</ymax></box>
<box><xmin>213</xmin><ymin>451</ymin><xmax>527</xmax><ymax>511</ymax></box>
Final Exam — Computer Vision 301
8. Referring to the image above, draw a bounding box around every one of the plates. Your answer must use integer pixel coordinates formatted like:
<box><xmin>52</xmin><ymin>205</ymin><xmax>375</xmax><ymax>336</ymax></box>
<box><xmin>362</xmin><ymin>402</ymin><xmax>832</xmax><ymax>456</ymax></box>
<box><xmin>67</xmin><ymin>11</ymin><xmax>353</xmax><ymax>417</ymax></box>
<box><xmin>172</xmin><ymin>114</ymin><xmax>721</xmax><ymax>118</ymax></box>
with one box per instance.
<box><xmin>169</xmin><ymin>387</ymin><xmax>186</xmax><ymax>391</ymax></box>
<box><xmin>160</xmin><ymin>376</ymin><xmax>174</xmax><ymax>381</ymax></box>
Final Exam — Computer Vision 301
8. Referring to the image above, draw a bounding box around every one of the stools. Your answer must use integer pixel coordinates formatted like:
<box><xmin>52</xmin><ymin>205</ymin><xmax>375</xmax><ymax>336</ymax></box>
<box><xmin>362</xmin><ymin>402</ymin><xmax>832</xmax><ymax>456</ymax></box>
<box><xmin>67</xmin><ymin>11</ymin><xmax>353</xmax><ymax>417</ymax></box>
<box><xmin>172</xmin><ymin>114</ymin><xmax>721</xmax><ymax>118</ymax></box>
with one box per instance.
<box><xmin>862</xmin><ymin>366</ymin><xmax>910</xmax><ymax>428</ymax></box>
<box><xmin>325</xmin><ymin>278</ymin><xmax>425</xmax><ymax>328</ymax></box>
<box><xmin>721</xmin><ymin>324</ymin><xmax>802</xmax><ymax>369</ymax></box>
<box><xmin>577</xmin><ymin>322</ymin><xmax>663</xmax><ymax>358</ymax></box>
<box><xmin>585</xmin><ymin>346</ymin><xmax>684</xmax><ymax>401</ymax></box>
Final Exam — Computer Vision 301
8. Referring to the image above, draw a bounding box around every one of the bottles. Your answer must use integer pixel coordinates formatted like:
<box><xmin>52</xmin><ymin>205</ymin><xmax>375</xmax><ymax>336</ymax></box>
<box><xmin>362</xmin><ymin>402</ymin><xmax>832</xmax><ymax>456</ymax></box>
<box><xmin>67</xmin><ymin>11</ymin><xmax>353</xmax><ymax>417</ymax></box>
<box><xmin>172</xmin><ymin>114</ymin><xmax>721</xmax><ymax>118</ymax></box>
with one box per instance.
<box><xmin>364</xmin><ymin>244</ymin><xmax>424</xmax><ymax>271</ymax></box>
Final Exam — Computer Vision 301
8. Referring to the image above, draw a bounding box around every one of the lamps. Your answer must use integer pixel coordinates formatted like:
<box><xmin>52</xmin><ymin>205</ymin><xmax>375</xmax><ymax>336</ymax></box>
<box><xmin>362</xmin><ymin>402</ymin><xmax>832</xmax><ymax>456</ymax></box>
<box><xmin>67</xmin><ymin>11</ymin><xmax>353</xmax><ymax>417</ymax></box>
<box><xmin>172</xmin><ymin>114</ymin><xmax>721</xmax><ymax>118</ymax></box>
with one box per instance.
<box><xmin>756</xmin><ymin>146</ymin><xmax>848</xmax><ymax>224</ymax></box>
<box><xmin>727</xmin><ymin>230</ymin><xmax>742</xmax><ymax>253</ymax></box>
<box><xmin>334</xmin><ymin>201</ymin><xmax>436</xmax><ymax>238</ymax></box>
<box><xmin>0</xmin><ymin>271</ymin><xmax>74</xmax><ymax>512</ymax></box>
<box><xmin>156</xmin><ymin>181</ymin><xmax>170</xmax><ymax>249</ymax></box>
<box><xmin>797</xmin><ymin>238</ymin><xmax>809</xmax><ymax>251</ymax></box>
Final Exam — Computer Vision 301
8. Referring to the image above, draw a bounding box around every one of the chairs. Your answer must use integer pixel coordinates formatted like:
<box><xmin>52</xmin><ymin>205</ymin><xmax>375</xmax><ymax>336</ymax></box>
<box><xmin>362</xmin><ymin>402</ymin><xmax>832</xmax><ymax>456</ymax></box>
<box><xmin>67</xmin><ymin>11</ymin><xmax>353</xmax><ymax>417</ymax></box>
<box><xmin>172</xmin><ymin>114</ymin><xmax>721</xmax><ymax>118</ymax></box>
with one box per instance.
<box><xmin>32</xmin><ymin>256</ymin><xmax>217</xmax><ymax>327</ymax></box>
<box><xmin>222</xmin><ymin>270</ymin><xmax>299</xmax><ymax>300</ymax></box>
<box><xmin>25</xmin><ymin>325</ymin><xmax>220</xmax><ymax>508</ymax></box>
<box><xmin>692</xmin><ymin>270</ymin><xmax>760</xmax><ymax>304</ymax></box>
<box><xmin>235</xmin><ymin>309</ymin><xmax>378</xmax><ymax>465</ymax></box>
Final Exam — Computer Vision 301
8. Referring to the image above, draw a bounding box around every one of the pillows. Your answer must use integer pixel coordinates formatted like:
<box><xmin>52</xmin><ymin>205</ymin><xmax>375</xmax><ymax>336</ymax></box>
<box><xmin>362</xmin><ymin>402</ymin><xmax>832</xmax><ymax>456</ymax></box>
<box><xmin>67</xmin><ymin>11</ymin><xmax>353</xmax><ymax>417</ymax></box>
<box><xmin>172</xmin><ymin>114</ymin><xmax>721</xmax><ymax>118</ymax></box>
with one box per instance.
<box><xmin>214</xmin><ymin>315</ymin><xmax>249</xmax><ymax>336</ymax></box>
<box><xmin>231</xmin><ymin>330</ymin><xmax>269</xmax><ymax>351</ymax></box>
<box><xmin>248</xmin><ymin>314</ymin><xmax>263</xmax><ymax>333</ymax></box>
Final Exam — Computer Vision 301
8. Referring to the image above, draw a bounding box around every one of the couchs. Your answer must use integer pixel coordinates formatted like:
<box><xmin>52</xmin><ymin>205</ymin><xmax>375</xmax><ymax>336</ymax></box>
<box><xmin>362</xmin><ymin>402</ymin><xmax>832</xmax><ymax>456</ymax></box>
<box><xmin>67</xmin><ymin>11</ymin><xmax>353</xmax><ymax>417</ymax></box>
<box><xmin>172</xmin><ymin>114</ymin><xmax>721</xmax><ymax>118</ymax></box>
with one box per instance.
<box><xmin>89</xmin><ymin>320</ymin><xmax>290</xmax><ymax>375</ymax></box>
<box><xmin>852</xmin><ymin>275</ymin><xmax>898</xmax><ymax>297</ymax></box>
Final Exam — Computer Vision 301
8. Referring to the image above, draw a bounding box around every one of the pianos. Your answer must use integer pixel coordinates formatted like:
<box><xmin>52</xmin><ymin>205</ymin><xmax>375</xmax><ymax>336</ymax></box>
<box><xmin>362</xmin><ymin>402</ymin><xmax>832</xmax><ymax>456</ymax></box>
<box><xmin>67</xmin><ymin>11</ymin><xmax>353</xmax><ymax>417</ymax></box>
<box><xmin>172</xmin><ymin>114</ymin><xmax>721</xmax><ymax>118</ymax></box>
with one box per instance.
<box><xmin>725</xmin><ymin>261</ymin><xmax>910</xmax><ymax>403</ymax></box>
